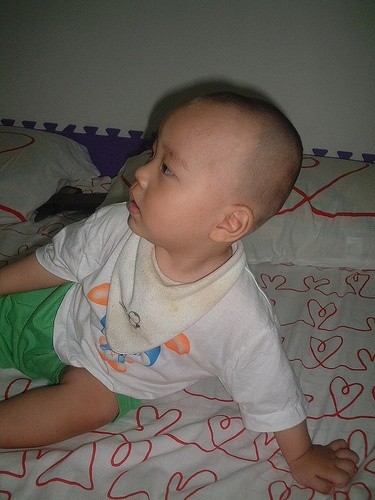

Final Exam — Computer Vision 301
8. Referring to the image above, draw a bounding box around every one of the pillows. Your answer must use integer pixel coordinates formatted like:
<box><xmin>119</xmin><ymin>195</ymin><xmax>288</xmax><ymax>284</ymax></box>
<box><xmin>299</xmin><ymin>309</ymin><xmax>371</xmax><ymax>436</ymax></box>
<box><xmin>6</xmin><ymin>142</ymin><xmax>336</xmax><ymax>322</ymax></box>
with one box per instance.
<box><xmin>96</xmin><ymin>155</ymin><xmax>375</xmax><ymax>270</ymax></box>
<box><xmin>0</xmin><ymin>127</ymin><xmax>98</xmax><ymax>225</ymax></box>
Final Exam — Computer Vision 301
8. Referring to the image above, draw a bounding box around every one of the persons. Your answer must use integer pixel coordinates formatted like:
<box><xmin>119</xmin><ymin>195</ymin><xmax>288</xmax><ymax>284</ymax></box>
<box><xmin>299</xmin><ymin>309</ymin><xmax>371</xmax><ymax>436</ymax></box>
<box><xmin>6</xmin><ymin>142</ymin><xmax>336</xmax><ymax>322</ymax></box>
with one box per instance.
<box><xmin>0</xmin><ymin>92</ymin><xmax>359</xmax><ymax>494</ymax></box>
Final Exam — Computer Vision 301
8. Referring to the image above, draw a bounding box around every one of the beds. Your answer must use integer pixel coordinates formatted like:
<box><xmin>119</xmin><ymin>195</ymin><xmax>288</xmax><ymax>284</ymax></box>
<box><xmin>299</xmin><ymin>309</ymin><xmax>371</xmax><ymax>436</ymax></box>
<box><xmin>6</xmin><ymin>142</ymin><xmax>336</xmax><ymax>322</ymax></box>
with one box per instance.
<box><xmin>0</xmin><ymin>175</ymin><xmax>375</xmax><ymax>500</ymax></box>
